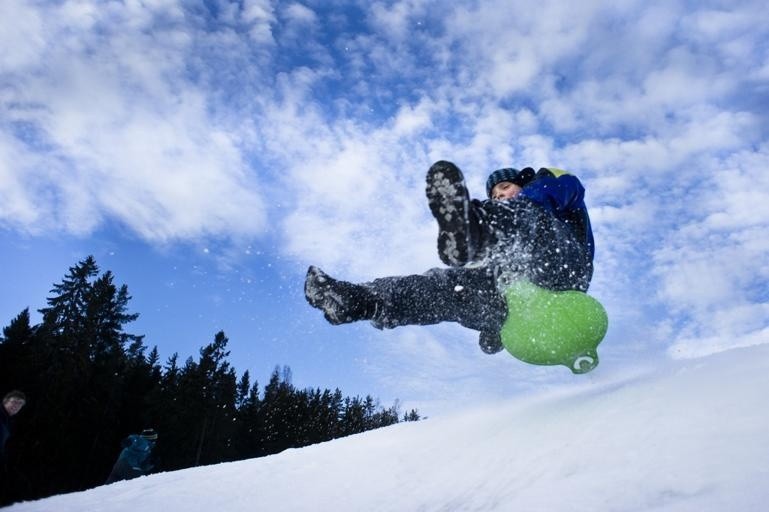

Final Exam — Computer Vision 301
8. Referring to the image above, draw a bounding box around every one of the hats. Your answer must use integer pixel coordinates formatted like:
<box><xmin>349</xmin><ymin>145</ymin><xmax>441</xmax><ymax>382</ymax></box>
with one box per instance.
<box><xmin>486</xmin><ymin>169</ymin><xmax>520</xmax><ymax>198</ymax></box>
<box><xmin>140</xmin><ymin>429</ymin><xmax>158</xmax><ymax>441</ymax></box>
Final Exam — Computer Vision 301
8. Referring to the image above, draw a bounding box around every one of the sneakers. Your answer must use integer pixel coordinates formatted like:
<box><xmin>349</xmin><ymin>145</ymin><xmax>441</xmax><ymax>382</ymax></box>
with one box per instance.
<box><xmin>304</xmin><ymin>266</ymin><xmax>380</xmax><ymax>325</ymax></box>
<box><xmin>425</xmin><ymin>161</ymin><xmax>481</xmax><ymax>267</ymax></box>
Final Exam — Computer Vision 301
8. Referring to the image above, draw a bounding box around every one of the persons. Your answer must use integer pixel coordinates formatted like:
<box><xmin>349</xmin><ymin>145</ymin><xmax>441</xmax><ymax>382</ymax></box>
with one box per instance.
<box><xmin>303</xmin><ymin>160</ymin><xmax>595</xmax><ymax>353</ymax></box>
<box><xmin>105</xmin><ymin>418</ymin><xmax>160</xmax><ymax>484</ymax></box>
<box><xmin>0</xmin><ymin>389</ymin><xmax>28</xmax><ymax>466</ymax></box>
